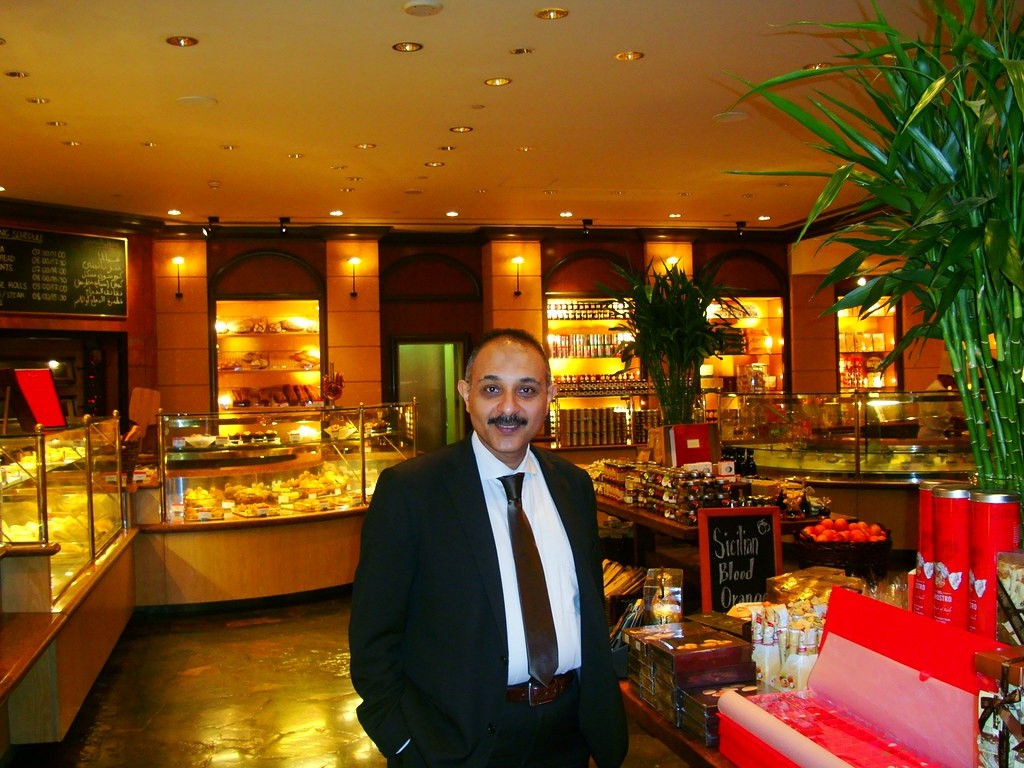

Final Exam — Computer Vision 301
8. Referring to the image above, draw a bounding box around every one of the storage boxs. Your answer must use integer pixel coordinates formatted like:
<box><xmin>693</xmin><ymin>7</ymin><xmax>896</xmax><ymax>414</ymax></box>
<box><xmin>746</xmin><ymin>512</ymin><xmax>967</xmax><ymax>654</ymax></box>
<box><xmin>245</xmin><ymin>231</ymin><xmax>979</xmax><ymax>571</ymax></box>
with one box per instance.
<box><xmin>620</xmin><ymin>565</ymin><xmax>783</xmax><ymax>749</ymax></box>
<box><xmin>974</xmin><ymin>547</ymin><xmax>1024</xmax><ymax>768</ymax></box>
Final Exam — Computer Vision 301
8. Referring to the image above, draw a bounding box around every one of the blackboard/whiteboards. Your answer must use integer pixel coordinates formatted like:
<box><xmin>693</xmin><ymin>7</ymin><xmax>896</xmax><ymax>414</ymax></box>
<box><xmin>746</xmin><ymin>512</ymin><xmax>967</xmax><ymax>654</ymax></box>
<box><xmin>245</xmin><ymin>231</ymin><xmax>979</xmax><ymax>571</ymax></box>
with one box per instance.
<box><xmin>0</xmin><ymin>226</ymin><xmax>129</xmax><ymax>319</ymax></box>
<box><xmin>698</xmin><ymin>505</ymin><xmax>783</xmax><ymax>613</ymax></box>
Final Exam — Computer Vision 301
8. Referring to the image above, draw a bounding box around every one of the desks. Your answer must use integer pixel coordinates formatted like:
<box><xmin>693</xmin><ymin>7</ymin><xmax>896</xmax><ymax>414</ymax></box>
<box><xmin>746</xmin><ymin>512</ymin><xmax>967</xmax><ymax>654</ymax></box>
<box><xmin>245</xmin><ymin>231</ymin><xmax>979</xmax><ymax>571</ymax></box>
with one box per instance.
<box><xmin>594</xmin><ymin>494</ymin><xmax>859</xmax><ymax>615</ymax></box>
<box><xmin>619</xmin><ymin>681</ymin><xmax>737</xmax><ymax>768</ymax></box>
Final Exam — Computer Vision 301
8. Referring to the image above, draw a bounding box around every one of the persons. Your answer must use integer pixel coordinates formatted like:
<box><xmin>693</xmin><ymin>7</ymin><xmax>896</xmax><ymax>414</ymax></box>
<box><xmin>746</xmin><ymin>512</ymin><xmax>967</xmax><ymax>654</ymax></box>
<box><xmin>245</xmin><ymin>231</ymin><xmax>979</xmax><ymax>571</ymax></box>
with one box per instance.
<box><xmin>348</xmin><ymin>329</ymin><xmax>627</xmax><ymax>768</ymax></box>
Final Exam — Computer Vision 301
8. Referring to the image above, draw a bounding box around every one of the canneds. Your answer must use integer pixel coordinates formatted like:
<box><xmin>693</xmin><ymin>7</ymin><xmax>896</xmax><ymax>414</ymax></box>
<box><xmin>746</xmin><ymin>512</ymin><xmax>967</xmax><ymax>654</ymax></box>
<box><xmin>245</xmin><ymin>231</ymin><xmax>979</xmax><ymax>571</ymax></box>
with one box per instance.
<box><xmin>693</xmin><ymin>408</ymin><xmax>718</xmax><ymax>424</ymax></box>
<box><xmin>552</xmin><ymin>373</ymin><xmax>653</xmax><ymax>396</ymax></box>
<box><xmin>546</xmin><ymin>303</ymin><xmax>631</xmax><ymax>319</ymax></box>
<box><xmin>558</xmin><ymin>408</ymin><xmax>660</xmax><ymax>446</ymax></box>
<box><xmin>603</xmin><ymin>460</ymin><xmax>773</xmax><ymax>528</ymax></box>
<box><xmin>547</xmin><ymin>333</ymin><xmax>621</xmax><ymax>358</ymax></box>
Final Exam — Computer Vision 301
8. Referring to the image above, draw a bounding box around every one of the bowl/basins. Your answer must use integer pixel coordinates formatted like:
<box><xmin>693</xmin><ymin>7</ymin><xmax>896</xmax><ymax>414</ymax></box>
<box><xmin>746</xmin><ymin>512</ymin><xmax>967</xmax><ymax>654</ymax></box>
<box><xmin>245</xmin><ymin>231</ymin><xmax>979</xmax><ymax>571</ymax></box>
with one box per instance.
<box><xmin>186</xmin><ymin>496</ymin><xmax>217</xmax><ymax>509</ymax></box>
<box><xmin>184</xmin><ymin>436</ymin><xmax>218</xmax><ymax>448</ymax></box>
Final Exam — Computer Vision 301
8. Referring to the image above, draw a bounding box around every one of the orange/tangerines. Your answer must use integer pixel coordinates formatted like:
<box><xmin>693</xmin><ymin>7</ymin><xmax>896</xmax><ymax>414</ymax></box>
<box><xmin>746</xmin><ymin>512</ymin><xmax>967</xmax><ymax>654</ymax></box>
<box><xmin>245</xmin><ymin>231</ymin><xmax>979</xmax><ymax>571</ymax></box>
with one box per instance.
<box><xmin>797</xmin><ymin>518</ymin><xmax>886</xmax><ymax>543</ymax></box>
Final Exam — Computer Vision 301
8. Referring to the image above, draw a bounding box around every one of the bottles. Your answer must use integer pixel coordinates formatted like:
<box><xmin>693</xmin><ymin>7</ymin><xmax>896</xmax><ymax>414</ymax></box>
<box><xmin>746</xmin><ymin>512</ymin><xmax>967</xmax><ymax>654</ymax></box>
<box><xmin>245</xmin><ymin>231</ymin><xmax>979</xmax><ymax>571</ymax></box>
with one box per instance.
<box><xmin>550</xmin><ymin>373</ymin><xmax>636</xmax><ymax>391</ymax></box>
<box><xmin>723</xmin><ymin>447</ymin><xmax>757</xmax><ymax>477</ymax></box>
<box><xmin>839</xmin><ymin>330</ymin><xmax>886</xmax><ymax>353</ymax></box>
<box><xmin>172</xmin><ymin>437</ymin><xmax>186</xmax><ymax>452</ymax></box>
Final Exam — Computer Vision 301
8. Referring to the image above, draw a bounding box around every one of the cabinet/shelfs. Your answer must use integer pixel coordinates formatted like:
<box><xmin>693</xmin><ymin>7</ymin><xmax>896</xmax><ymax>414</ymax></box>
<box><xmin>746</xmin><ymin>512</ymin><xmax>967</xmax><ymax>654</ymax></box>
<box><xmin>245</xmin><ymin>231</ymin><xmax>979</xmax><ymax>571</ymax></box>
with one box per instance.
<box><xmin>836</xmin><ymin>293</ymin><xmax>905</xmax><ymax>420</ymax></box>
<box><xmin>698</xmin><ymin>294</ymin><xmax>792</xmax><ymax>434</ymax></box>
<box><xmin>544</xmin><ymin>292</ymin><xmax>645</xmax><ymax>434</ymax></box>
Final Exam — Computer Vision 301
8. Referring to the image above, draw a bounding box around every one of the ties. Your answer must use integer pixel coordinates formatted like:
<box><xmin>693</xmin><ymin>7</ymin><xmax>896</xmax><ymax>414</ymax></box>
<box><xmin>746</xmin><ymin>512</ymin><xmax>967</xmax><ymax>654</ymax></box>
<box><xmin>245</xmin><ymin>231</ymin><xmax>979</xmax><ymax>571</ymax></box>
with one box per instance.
<box><xmin>497</xmin><ymin>473</ymin><xmax>559</xmax><ymax>687</ymax></box>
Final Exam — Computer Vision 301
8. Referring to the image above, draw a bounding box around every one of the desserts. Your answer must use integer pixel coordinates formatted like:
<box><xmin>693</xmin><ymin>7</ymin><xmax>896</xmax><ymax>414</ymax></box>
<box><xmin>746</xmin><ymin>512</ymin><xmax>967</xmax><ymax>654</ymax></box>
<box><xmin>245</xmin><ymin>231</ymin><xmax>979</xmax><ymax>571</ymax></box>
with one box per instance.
<box><xmin>227</xmin><ymin>429</ymin><xmax>278</xmax><ymax>443</ymax></box>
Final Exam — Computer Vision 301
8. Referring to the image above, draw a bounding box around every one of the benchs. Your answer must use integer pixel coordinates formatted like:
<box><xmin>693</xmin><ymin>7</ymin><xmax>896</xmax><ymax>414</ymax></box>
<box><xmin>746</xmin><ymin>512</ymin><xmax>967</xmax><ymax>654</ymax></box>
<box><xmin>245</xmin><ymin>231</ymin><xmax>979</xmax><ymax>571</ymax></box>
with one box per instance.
<box><xmin>215</xmin><ymin>294</ymin><xmax>323</xmax><ymax>441</ymax></box>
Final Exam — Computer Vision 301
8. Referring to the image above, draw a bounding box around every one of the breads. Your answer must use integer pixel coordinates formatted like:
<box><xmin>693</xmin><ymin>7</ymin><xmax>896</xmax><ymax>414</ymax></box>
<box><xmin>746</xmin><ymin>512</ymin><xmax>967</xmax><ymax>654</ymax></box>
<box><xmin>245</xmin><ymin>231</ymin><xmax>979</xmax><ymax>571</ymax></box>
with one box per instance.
<box><xmin>2</xmin><ymin>514</ymin><xmax>114</xmax><ymax>555</ymax></box>
<box><xmin>183</xmin><ymin>463</ymin><xmax>378</xmax><ymax>520</ymax></box>
<box><xmin>0</xmin><ymin>447</ymin><xmax>86</xmax><ymax>484</ymax></box>
<box><xmin>217</xmin><ymin>319</ymin><xmax>324</xmax><ymax>407</ymax></box>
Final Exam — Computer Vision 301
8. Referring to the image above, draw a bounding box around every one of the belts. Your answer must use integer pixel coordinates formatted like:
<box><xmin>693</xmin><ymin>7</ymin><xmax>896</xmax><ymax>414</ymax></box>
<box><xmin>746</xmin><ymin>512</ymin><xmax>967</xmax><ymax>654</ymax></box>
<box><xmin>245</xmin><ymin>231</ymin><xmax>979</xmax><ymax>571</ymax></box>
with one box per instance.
<box><xmin>506</xmin><ymin>670</ymin><xmax>570</xmax><ymax>706</ymax></box>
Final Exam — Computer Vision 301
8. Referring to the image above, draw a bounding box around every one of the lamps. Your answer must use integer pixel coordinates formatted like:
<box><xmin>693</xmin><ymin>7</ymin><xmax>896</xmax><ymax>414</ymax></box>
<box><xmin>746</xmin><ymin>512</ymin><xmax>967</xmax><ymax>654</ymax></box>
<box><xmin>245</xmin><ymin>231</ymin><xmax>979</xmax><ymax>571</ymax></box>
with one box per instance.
<box><xmin>280</xmin><ymin>217</ymin><xmax>291</xmax><ymax>232</ymax></box>
<box><xmin>582</xmin><ymin>219</ymin><xmax>592</xmax><ymax>235</ymax></box>
<box><xmin>737</xmin><ymin>221</ymin><xmax>746</xmax><ymax>236</ymax></box>
<box><xmin>202</xmin><ymin>215</ymin><xmax>219</xmax><ymax>236</ymax></box>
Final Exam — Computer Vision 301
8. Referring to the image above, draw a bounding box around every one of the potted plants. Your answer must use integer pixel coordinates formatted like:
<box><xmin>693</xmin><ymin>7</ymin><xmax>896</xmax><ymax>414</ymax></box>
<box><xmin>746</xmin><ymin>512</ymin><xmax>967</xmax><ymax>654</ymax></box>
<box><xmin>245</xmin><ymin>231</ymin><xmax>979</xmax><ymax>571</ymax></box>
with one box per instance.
<box><xmin>715</xmin><ymin>0</ymin><xmax>1024</xmax><ymax>551</ymax></box>
<box><xmin>594</xmin><ymin>251</ymin><xmax>750</xmax><ymax>470</ymax></box>
